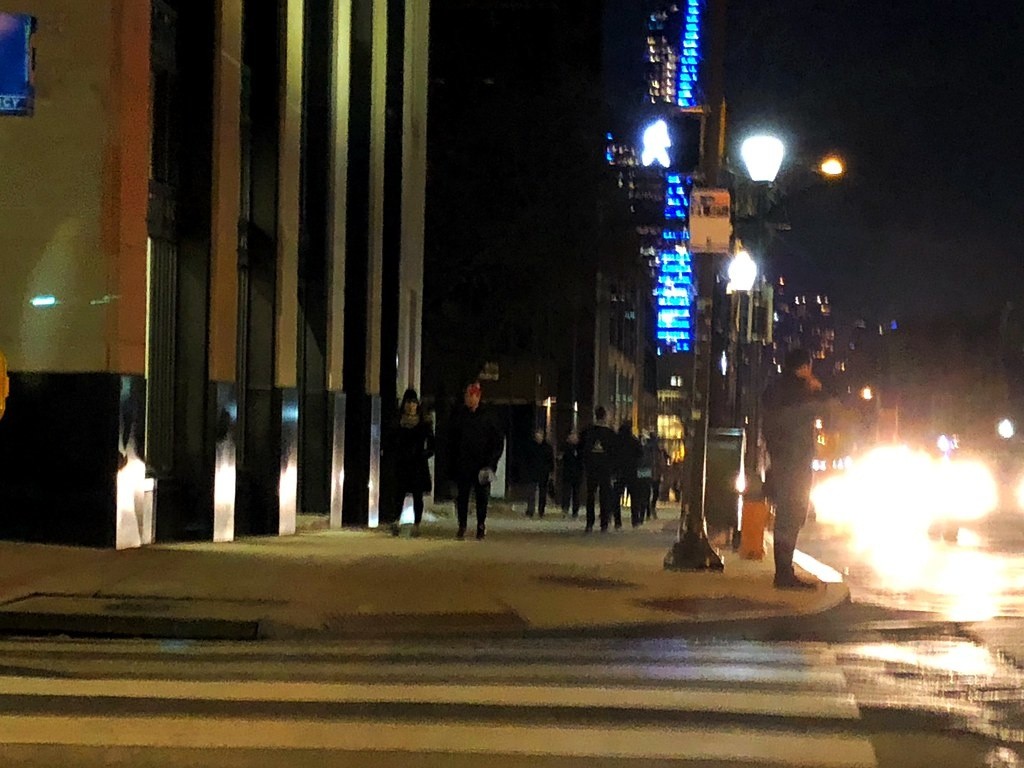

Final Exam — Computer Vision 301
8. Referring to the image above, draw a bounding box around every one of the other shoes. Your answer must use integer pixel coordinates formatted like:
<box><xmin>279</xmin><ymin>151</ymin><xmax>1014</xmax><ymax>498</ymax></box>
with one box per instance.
<box><xmin>774</xmin><ymin>574</ymin><xmax>817</xmax><ymax>591</ymax></box>
<box><xmin>410</xmin><ymin>526</ymin><xmax>421</xmax><ymax>538</ymax></box>
<box><xmin>476</xmin><ymin>524</ymin><xmax>486</xmax><ymax>540</ymax></box>
<box><xmin>601</xmin><ymin>528</ymin><xmax>608</xmax><ymax>535</ymax></box>
<box><xmin>537</xmin><ymin>512</ymin><xmax>544</xmax><ymax>519</ymax></box>
<box><xmin>392</xmin><ymin>525</ymin><xmax>399</xmax><ymax>537</ymax></box>
<box><xmin>455</xmin><ymin>526</ymin><xmax>466</xmax><ymax>538</ymax></box>
<box><xmin>526</xmin><ymin>511</ymin><xmax>532</xmax><ymax>517</ymax></box>
<box><xmin>585</xmin><ymin>527</ymin><xmax>593</xmax><ymax>534</ymax></box>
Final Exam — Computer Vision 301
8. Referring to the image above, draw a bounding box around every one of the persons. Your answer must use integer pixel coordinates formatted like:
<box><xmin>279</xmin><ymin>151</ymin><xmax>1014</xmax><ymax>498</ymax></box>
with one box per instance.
<box><xmin>621</xmin><ymin>429</ymin><xmax>684</xmax><ymax>518</ymax></box>
<box><xmin>754</xmin><ymin>347</ymin><xmax>833</xmax><ymax>591</ymax></box>
<box><xmin>556</xmin><ymin>432</ymin><xmax>587</xmax><ymax>519</ymax></box>
<box><xmin>576</xmin><ymin>406</ymin><xmax>620</xmax><ymax>533</ymax></box>
<box><xmin>445</xmin><ymin>382</ymin><xmax>504</xmax><ymax>540</ymax></box>
<box><xmin>520</xmin><ymin>426</ymin><xmax>554</xmax><ymax>519</ymax></box>
<box><xmin>610</xmin><ymin>422</ymin><xmax>644</xmax><ymax>530</ymax></box>
<box><xmin>379</xmin><ymin>387</ymin><xmax>436</xmax><ymax>536</ymax></box>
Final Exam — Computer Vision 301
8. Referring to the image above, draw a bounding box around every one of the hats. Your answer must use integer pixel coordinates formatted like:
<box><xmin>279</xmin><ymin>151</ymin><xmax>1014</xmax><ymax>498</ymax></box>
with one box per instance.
<box><xmin>403</xmin><ymin>388</ymin><xmax>418</xmax><ymax>400</ymax></box>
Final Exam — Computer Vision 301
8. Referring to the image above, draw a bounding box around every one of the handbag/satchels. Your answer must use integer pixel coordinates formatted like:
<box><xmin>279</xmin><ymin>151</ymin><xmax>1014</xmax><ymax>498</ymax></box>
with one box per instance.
<box><xmin>765</xmin><ymin>465</ymin><xmax>777</xmax><ymax>501</ymax></box>
<box><xmin>637</xmin><ymin>459</ymin><xmax>653</xmax><ymax>480</ymax></box>
